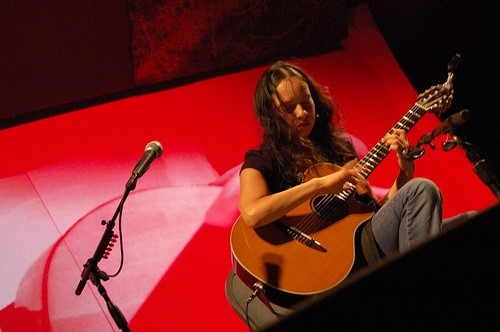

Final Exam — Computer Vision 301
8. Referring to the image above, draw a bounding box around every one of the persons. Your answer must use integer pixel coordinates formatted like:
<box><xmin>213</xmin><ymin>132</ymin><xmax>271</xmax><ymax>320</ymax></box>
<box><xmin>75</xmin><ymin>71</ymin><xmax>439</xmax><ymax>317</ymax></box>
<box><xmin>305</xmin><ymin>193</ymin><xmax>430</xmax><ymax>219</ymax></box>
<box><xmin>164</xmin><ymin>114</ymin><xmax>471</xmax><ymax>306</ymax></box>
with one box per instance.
<box><xmin>239</xmin><ymin>61</ymin><xmax>481</xmax><ymax>271</ymax></box>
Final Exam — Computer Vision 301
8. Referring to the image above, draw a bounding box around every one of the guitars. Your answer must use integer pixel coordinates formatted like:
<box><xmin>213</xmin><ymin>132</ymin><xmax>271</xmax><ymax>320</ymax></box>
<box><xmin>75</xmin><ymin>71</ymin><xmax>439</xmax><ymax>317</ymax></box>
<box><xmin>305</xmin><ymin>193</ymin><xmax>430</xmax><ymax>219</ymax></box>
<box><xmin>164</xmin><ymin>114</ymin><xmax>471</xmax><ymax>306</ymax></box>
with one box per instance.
<box><xmin>228</xmin><ymin>75</ymin><xmax>457</xmax><ymax>300</ymax></box>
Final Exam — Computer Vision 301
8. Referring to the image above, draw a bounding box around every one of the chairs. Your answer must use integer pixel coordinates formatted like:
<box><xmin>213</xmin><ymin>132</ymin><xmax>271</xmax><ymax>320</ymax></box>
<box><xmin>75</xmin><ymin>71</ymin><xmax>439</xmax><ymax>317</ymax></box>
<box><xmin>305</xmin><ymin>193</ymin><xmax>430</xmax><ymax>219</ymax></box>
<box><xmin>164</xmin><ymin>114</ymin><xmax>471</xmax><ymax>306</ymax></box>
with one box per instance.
<box><xmin>226</xmin><ymin>271</ymin><xmax>290</xmax><ymax>332</ymax></box>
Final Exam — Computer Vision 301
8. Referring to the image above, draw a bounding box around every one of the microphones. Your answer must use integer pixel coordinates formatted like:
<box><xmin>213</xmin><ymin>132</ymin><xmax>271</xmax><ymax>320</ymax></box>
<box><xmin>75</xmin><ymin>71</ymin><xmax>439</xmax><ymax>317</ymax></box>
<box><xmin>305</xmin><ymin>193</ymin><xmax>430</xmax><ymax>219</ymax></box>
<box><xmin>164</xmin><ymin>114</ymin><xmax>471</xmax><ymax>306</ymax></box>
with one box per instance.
<box><xmin>402</xmin><ymin>147</ymin><xmax>425</xmax><ymax>160</ymax></box>
<box><xmin>126</xmin><ymin>140</ymin><xmax>163</xmax><ymax>190</ymax></box>
